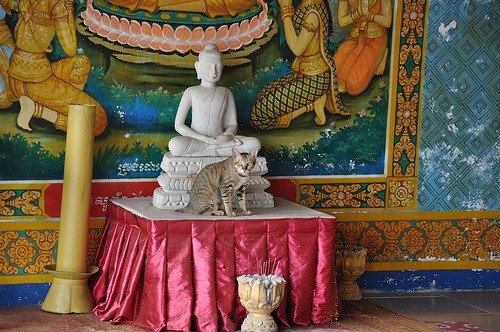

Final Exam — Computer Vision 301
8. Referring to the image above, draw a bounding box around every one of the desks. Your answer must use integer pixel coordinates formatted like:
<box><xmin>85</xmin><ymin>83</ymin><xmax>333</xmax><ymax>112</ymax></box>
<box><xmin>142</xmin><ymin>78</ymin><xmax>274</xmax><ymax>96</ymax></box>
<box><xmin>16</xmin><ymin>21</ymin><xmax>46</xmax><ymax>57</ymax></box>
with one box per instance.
<box><xmin>91</xmin><ymin>197</ymin><xmax>340</xmax><ymax>332</ymax></box>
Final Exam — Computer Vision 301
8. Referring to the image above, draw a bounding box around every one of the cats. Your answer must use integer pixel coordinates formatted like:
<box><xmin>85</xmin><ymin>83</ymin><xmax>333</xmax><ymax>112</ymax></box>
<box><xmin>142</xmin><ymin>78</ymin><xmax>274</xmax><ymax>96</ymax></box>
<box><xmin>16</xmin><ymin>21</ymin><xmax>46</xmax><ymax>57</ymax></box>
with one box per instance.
<box><xmin>174</xmin><ymin>146</ymin><xmax>258</xmax><ymax>217</ymax></box>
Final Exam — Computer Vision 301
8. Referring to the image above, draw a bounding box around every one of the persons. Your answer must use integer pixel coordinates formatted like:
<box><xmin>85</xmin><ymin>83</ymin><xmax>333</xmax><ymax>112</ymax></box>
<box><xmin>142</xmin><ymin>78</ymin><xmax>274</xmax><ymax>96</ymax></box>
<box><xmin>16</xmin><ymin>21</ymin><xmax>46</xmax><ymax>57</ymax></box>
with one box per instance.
<box><xmin>167</xmin><ymin>44</ymin><xmax>262</xmax><ymax>156</ymax></box>
